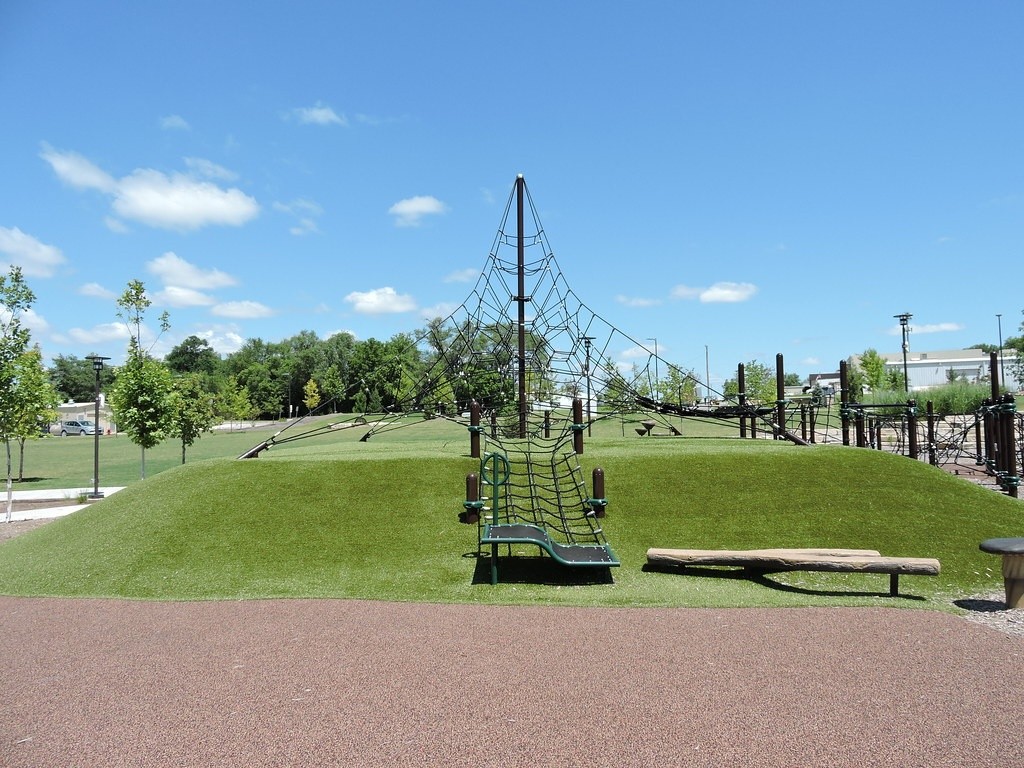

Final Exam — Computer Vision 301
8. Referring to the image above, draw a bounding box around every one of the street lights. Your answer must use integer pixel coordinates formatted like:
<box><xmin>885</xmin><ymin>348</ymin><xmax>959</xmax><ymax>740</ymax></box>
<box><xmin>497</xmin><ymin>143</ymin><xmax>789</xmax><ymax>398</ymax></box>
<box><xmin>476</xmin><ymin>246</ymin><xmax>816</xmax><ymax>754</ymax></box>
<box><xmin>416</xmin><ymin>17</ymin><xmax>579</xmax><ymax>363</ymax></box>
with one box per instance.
<box><xmin>996</xmin><ymin>314</ymin><xmax>1005</xmax><ymax>388</ymax></box>
<box><xmin>893</xmin><ymin>313</ymin><xmax>913</xmax><ymax>391</ymax></box>
<box><xmin>579</xmin><ymin>337</ymin><xmax>596</xmax><ymax>438</ymax></box>
<box><xmin>86</xmin><ymin>356</ymin><xmax>111</xmax><ymax>498</ymax></box>
<box><xmin>647</xmin><ymin>338</ymin><xmax>659</xmax><ymax>400</ymax></box>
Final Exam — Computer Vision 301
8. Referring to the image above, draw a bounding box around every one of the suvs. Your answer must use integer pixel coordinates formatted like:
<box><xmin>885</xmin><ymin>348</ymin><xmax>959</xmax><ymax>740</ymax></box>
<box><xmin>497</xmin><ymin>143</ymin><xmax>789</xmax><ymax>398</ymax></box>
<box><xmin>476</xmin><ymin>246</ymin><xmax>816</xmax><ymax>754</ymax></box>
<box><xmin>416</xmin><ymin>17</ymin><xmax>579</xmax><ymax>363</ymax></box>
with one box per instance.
<box><xmin>60</xmin><ymin>420</ymin><xmax>105</xmax><ymax>437</ymax></box>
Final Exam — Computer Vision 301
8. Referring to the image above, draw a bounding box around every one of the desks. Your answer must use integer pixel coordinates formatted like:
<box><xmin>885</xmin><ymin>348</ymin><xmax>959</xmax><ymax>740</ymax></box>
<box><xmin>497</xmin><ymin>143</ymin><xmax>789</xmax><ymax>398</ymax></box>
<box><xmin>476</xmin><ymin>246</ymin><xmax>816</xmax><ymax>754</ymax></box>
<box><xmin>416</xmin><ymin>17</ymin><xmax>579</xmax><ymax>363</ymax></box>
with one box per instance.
<box><xmin>980</xmin><ymin>537</ymin><xmax>1024</xmax><ymax>610</ymax></box>
